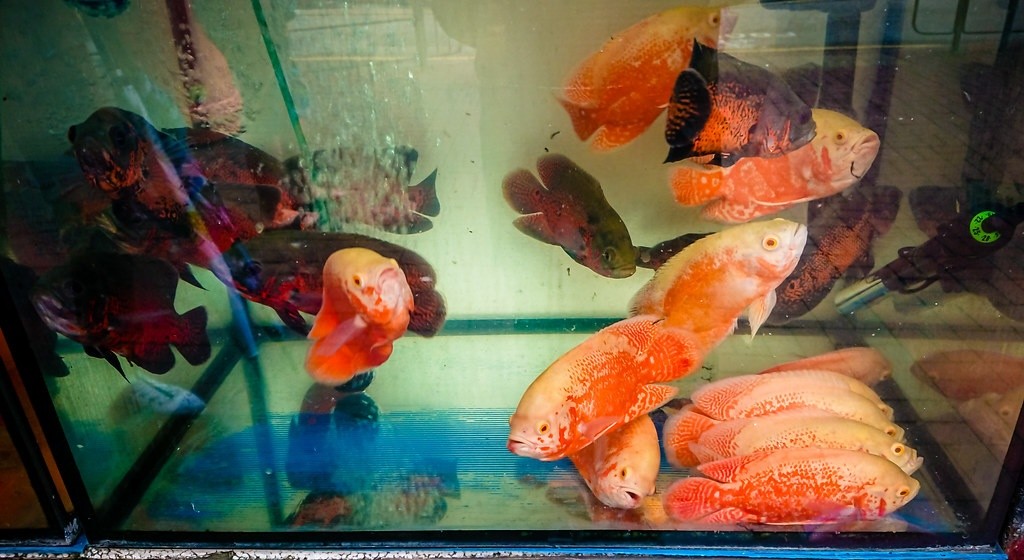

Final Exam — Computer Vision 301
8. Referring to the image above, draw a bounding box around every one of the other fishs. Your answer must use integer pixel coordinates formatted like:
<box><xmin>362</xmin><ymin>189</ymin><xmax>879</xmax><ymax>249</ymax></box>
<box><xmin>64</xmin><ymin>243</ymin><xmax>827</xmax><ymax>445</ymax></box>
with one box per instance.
<box><xmin>0</xmin><ymin>0</ymin><xmax>461</xmax><ymax>529</ymax></box>
<box><xmin>907</xmin><ymin>61</ymin><xmax>1024</xmax><ymax>452</ymax></box>
<box><xmin>501</xmin><ymin>7</ymin><xmax>924</xmax><ymax>529</ymax></box>
